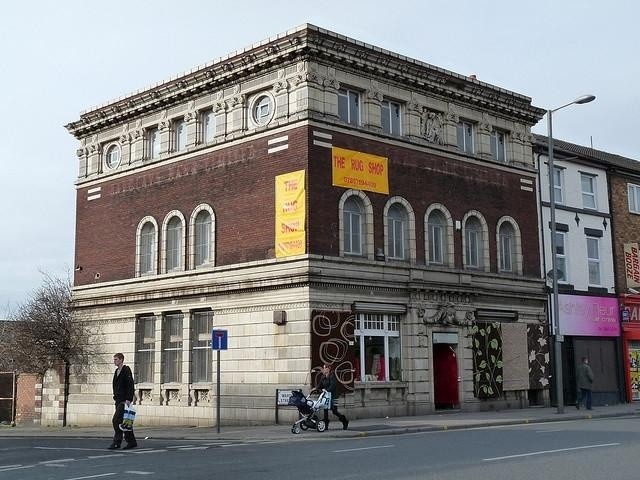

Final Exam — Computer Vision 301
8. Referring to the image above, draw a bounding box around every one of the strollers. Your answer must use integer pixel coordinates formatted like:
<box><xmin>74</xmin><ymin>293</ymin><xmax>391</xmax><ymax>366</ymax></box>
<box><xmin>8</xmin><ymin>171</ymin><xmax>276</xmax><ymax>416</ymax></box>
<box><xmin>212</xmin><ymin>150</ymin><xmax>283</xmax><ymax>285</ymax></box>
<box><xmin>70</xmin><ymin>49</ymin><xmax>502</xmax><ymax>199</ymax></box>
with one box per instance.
<box><xmin>290</xmin><ymin>387</ymin><xmax>326</xmax><ymax>435</ymax></box>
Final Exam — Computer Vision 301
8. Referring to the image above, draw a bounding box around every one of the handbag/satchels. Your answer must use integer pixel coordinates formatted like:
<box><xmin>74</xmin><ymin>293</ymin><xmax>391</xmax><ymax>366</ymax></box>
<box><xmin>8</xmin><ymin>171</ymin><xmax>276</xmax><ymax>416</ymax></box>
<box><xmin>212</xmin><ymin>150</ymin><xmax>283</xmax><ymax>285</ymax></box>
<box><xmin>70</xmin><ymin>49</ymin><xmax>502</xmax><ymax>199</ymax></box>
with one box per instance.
<box><xmin>323</xmin><ymin>392</ymin><xmax>332</xmax><ymax>409</ymax></box>
<box><xmin>119</xmin><ymin>403</ymin><xmax>136</xmax><ymax>432</ymax></box>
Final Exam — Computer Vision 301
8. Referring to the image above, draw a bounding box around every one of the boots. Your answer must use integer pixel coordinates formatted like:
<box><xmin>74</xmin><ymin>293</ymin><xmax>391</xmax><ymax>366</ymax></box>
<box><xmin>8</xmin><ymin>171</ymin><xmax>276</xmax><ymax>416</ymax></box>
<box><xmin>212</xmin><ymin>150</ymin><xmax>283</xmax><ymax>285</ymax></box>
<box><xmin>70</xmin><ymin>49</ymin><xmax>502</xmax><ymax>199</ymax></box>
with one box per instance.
<box><xmin>323</xmin><ymin>418</ymin><xmax>330</xmax><ymax>431</ymax></box>
<box><xmin>339</xmin><ymin>415</ymin><xmax>350</xmax><ymax>429</ymax></box>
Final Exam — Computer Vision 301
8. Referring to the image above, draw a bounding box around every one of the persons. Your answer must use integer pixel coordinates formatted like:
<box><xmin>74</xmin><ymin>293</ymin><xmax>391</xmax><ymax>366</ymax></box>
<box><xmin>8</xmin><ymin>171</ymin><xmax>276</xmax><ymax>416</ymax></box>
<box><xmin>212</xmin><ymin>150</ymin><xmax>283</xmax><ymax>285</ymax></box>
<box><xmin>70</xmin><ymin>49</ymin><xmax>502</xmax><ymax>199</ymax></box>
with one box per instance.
<box><xmin>315</xmin><ymin>364</ymin><xmax>349</xmax><ymax>430</ymax></box>
<box><xmin>575</xmin><ymin>356</ymin><xmax>594</xmax><ymax>411</ymax></box>
<box><xmin>106</xmin><ymin>352</ymin><xmax>138</xmax><ymax>449</ymax></box>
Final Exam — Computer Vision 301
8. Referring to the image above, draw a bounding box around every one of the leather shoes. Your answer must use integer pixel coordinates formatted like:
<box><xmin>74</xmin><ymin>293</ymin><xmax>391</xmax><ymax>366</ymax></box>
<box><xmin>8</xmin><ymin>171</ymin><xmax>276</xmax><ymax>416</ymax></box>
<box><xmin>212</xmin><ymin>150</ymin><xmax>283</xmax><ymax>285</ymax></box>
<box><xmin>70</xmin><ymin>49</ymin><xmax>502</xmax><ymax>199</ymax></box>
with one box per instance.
<box><xmin>108</xmin><ymin>443</ymin><xmax>122</xmax><ymax>450</ymax></box>
<box><xmin>124</xmin><ymin>442</ymin><xmax>138</xmax><ymax>449</ymax></box>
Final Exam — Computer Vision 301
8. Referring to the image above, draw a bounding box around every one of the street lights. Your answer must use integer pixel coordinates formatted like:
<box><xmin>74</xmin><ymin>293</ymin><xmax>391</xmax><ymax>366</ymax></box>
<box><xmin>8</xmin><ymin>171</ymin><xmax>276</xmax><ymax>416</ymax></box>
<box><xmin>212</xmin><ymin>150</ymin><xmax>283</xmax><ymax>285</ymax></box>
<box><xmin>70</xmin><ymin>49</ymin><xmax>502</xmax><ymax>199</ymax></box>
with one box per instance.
<box><xmin>547</xmin><ymin>94</ymin><xmax>596</xmax><ymax>414</ymax></box>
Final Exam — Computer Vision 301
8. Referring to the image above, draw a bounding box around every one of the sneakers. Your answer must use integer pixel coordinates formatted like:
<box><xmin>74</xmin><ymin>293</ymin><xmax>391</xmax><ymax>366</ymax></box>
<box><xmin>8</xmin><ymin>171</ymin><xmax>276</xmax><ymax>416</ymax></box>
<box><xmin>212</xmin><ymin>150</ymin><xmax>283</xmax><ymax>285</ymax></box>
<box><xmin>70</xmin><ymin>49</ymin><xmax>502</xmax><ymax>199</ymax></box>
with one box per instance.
<box><xmin>586</xmin><ymin>406</ymin><xmax>595</xmax><ymax>410</ymax></box>
<box><xmin>576</xmin><ymin>402</ymin><xmax>580</xmax><ymax>411</ymax></box>
